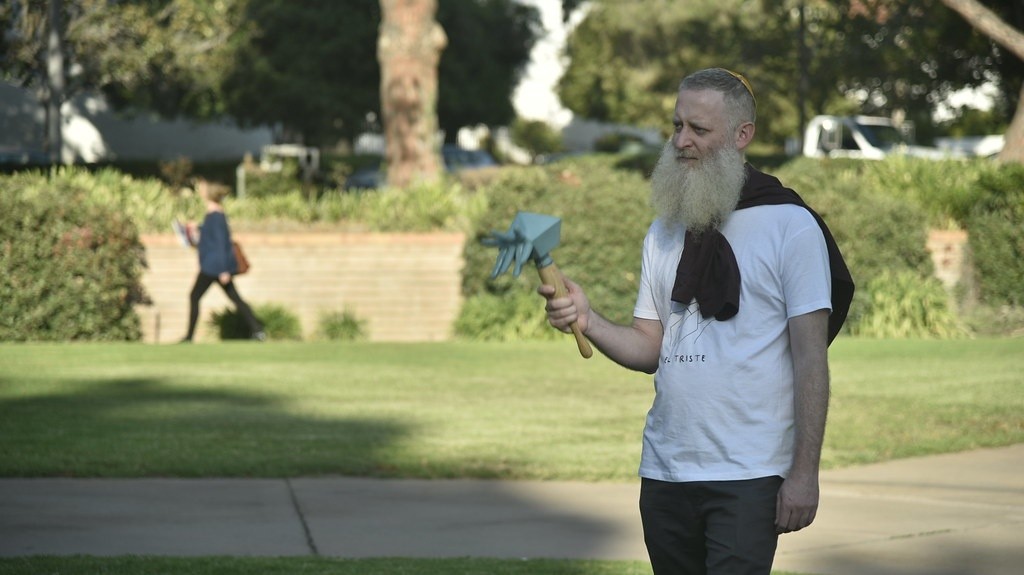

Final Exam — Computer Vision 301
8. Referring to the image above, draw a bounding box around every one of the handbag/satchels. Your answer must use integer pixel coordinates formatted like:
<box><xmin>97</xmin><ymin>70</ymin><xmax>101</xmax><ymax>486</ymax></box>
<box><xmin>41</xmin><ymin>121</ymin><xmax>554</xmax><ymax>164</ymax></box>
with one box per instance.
<box><xmin>230</xmin><ymin>242</ymin><xmax>248</xmax><ymax>275</ymax></box>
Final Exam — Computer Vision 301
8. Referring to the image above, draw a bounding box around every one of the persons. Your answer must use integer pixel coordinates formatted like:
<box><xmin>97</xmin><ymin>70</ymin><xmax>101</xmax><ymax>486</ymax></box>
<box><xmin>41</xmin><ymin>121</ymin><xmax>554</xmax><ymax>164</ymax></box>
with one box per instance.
<box><xmin>538</xmin><ymin>68</ymin><xmax>832</xmax><ymax>575</ymax></box>
<box><xmin>176</xmin><ymin>182</ymin><xmax>268</xmax><ymax>342</ymax></box>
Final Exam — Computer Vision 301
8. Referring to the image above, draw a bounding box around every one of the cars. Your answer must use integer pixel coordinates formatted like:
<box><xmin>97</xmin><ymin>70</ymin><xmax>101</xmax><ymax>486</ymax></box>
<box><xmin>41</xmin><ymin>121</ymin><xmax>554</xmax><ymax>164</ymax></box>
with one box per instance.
<box><xmin>795</xmin><ymin>110</ymin><xmax>966</xmax><ymax>166</ymax></box>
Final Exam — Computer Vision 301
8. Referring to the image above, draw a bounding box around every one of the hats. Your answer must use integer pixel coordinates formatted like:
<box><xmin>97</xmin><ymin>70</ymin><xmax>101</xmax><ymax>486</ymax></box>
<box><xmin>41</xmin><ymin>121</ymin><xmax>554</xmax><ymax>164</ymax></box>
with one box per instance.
<box><xmin>720</xmin><ymin>68</ymin><xmax>756</xmax><ymax>105</ymax></box>
